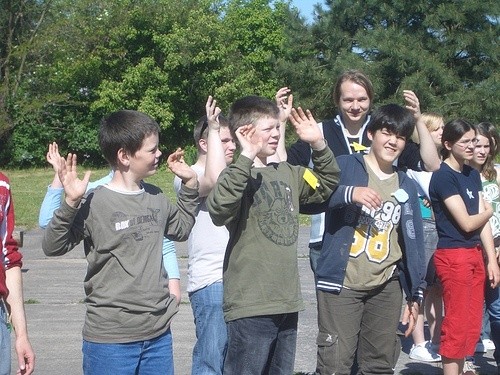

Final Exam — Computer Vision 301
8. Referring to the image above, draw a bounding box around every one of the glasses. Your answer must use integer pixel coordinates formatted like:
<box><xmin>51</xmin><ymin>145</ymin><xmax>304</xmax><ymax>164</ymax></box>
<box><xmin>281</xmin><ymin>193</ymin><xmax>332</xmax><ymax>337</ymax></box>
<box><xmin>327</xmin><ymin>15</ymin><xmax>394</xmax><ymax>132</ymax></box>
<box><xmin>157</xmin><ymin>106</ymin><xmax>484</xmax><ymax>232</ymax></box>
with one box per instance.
<box><xmin>454</xmin><ymin>139</ymin><xmax>480</xmax><ymax>146</ymax></box>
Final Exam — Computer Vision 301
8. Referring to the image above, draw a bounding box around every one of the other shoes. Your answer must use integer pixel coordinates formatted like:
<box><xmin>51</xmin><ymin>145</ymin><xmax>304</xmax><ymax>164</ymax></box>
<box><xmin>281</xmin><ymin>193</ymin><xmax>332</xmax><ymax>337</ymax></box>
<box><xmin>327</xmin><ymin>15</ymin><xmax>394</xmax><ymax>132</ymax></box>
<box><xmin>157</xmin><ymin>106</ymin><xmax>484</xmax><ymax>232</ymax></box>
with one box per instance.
<box><xmin>475</xmin><ymin>338</ymin><xmax>496</xmax><ymax>352</ymax></box>
<box><xmin>463</xmin><ymin>360</ymin><xmax>481</xmax><ymax>374</ymax></box>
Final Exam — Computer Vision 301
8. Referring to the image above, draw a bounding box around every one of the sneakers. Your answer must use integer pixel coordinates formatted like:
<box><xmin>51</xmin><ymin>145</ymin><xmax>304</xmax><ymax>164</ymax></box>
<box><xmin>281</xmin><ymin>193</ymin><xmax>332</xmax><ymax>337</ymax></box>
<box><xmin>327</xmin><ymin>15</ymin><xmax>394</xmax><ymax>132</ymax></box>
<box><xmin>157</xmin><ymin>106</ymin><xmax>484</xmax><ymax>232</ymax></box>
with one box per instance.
<box><xmin>408</xmin><ymin>341</ymin><xmax>442</xmax><ymax>362</ymax></box>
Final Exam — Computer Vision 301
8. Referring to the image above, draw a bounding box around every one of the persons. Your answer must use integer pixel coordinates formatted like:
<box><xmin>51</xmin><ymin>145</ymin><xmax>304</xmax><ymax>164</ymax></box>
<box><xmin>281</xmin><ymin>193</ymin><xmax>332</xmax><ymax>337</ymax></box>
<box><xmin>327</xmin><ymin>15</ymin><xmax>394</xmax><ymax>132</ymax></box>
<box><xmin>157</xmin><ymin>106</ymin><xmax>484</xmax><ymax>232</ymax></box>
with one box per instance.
<box><xmin>0</xmin><ymin>171</ymin><xmax>35</xmax><ymax>375</ymax></box>
<box><xmin>429</xmin><ymin>119</ymin><xmax>500</xmax><ymax>375</ymax></box>
<box><xmin>42</xmin><ymin>109</ymin><xmax>200</xmax><ymax>375</ymax></box>
<box><xmin>38</xmin><ymin>141</ymin><xmax>180</xmax><ymax>306</ymax></box>
<box><xmin>206</xmin><ymin>95</ymin><xmax>342</xmax><ymax>375</ymax></box>
<box><xmin>174</xmin><ymin>87</ymin><xmax>293</xmax><ymax>375</ymax></box>
<box><xmin>315</xmin><ymin>102</ymin><xmax>427</xmax><ymax>375</ymax></box>
<box><xmin>274</xmin><ymin>70</ymin><xmax>440</xmax><ymax>323</ymax></box>
<box><xmin>397</xmin><ymin>111</ymin><xmax>500</xmax><ymax>369</ymax></box>
